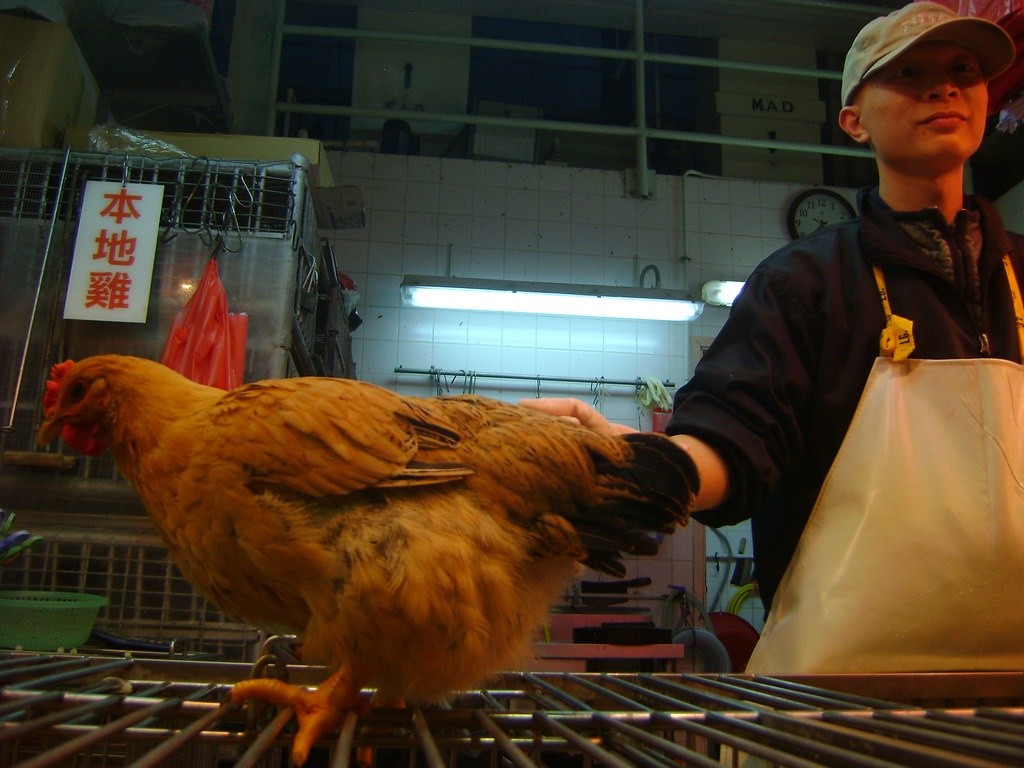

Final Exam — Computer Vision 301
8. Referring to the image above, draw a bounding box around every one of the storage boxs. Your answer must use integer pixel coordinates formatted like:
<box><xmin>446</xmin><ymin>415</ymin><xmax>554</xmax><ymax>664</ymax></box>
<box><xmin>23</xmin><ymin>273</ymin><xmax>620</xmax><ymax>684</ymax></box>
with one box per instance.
<box><xmin>0</xmin><ymin>5</ymin><xmax>102</xmax><ymax>149</ymax></box>
<box><xmin>63</xmin><ymin>124</ymin><xmax>366</xmax><ymax>231</ymax></box>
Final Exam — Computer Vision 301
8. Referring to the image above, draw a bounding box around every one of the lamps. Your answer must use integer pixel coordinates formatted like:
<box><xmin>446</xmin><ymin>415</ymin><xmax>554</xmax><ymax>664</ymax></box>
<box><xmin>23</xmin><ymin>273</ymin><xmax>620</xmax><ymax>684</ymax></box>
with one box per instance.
<box><xmin>701</xmin><ymin>280</ymin><xmax>747</xmax><ymax>307</ymax></box>
<box><xmin>401</xmin><ymin>264</ymin><xmax>706</xmax><ymax>322</ymax></box>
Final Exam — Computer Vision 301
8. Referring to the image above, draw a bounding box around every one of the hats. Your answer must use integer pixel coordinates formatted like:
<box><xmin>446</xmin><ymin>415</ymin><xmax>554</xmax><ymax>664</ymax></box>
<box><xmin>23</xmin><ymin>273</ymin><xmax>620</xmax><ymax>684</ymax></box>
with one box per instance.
<box><xmin>840</xmin><ymin>1</ymin><xmax>1017</xmax><ymax>106</ymax></box>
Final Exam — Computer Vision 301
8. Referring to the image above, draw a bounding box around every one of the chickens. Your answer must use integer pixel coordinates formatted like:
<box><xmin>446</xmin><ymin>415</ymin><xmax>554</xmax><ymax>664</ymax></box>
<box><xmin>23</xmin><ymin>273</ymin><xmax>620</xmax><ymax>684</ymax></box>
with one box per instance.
<box><xmin>39</xmin><ymin>351</ymin><xmax>703</xmax><ymax>767</ymax></box>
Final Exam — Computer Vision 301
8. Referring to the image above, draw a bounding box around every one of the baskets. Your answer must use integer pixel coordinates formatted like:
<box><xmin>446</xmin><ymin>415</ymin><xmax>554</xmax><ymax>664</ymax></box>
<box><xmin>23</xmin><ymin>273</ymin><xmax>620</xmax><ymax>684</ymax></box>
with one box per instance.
<box><xmin>0</xmin><ymin>592</ymin><xmax>111</xmax><ymax>650</ymax></box>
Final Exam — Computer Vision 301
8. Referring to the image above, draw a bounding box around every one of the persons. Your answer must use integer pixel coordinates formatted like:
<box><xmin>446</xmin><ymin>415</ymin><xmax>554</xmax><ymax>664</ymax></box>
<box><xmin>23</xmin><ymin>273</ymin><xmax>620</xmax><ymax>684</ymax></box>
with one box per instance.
<box><xmin>526</xmin><ymin>2</ymin><xmax>1024</xmax><ymax>768</ymax></box>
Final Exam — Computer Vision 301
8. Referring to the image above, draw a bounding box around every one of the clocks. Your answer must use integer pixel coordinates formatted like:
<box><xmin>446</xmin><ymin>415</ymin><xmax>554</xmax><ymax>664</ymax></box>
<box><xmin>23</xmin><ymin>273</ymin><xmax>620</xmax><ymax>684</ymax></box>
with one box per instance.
<box><xmin>787</xmin><ymin>188</ymin><xmax>857</xmax><ymax>241</ymax></box>
